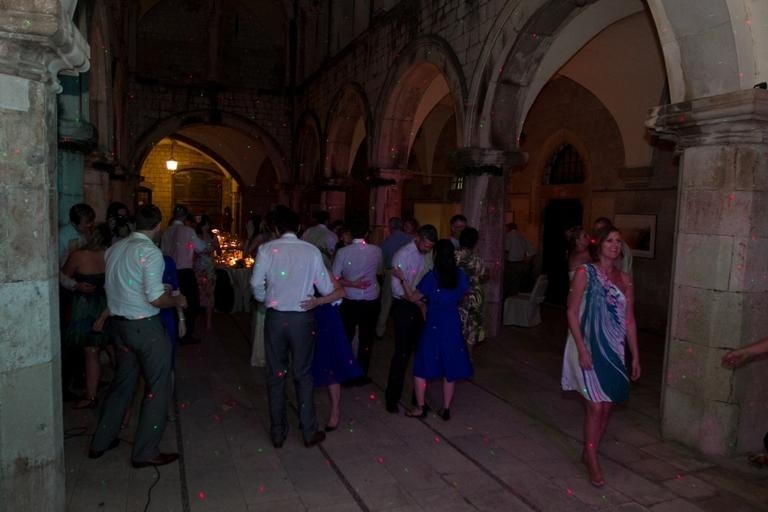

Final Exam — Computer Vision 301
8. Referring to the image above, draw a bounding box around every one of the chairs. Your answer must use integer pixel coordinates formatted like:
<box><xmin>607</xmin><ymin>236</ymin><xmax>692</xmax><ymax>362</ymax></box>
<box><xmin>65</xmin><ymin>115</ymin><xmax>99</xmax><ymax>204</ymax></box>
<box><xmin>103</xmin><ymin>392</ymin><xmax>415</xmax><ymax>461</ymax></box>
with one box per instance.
<box><xmin>501</xmin><ymin>272</ymin><xmax>551</xmax><ymax>331</ymax></box>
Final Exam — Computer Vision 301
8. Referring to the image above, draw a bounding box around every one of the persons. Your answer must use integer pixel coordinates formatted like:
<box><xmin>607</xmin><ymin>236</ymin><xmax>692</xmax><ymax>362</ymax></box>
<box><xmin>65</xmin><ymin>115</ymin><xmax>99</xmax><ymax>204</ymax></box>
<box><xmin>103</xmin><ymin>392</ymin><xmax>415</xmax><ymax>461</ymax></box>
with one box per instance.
<box><xmin>722</xmin><ymin>339</ymin><xmax>768</xmax><ymax>371</ymax></box>
<box><xmin>561</xmin><ymin>217</ymin><xmax>641</xmax><ymax>486</ymax></box>
<box><xmin>503</xmin><ymin>221</ymin><xmax>535</xmax><ymax>298</ymax></box>
<box><xmin>58</xmin><ymin>202</ymin><xmax>488</xmax><ymax>468</ymax></box>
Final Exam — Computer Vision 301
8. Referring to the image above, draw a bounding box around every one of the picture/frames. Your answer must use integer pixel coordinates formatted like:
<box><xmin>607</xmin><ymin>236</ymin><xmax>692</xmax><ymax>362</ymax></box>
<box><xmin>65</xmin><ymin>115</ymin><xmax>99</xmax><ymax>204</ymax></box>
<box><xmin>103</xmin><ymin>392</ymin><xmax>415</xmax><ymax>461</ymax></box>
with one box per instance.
<box><xmin>611</xmin><ymin>211</ymin><xmax>658</xmax><ymax>261</ymax></box>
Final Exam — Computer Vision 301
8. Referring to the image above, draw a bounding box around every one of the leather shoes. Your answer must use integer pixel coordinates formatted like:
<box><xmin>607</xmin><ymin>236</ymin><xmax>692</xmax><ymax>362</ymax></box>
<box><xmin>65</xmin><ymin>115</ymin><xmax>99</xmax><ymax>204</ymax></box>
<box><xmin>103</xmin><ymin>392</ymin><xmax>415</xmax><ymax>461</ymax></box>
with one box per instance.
<box><xmin>88</xmin><ymin>438</ymin><xmax>120</xmax><ymax>459</ymax></box>
<box><xmin>324</xmin><ymin>424</ymin><xmax>336</xmax><ymax>432</ymax></box>
<box><xmin>304</xmin><ymin>431</ymin><xmax>326</xmax><ymax>447</ymax></box>
<box><xmin>132</xmin><ymin>453</ymin><xmax>180</xmax><ymax>469</ymax></box>
<box><xmin>385</xmin><ymin>398</ymin><xmax>400</xmax><ymax>413</ymax></box>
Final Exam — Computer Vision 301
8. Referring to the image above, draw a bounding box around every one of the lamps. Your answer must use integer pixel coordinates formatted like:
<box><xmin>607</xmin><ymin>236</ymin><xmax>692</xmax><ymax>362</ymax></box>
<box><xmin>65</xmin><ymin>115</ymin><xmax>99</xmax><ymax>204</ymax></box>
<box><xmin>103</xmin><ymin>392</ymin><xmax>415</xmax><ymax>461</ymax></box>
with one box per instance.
<box><xmin>165</xmin><ymin>139</ymin><xmax>180</xmax><ymax>173</ymax></box>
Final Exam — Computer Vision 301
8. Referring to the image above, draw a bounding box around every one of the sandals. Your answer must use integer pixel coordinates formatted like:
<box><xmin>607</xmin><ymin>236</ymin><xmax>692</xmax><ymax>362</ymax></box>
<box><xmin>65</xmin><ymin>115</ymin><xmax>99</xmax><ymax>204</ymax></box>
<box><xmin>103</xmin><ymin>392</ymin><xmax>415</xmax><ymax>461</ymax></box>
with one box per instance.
<box><xmin>437</xmin><ymin>407</ymin><xmax>450</xmax><ymax>421</ymax></box>
<box><xmin>72</xmin><ymin>398</ymin><xmax>94</xmax><ymax>409</ymax></box>
<box><xmin>404</xmin><ymin>405</ymin><xmax>427</xmax><ymax>417</ymax></box>
